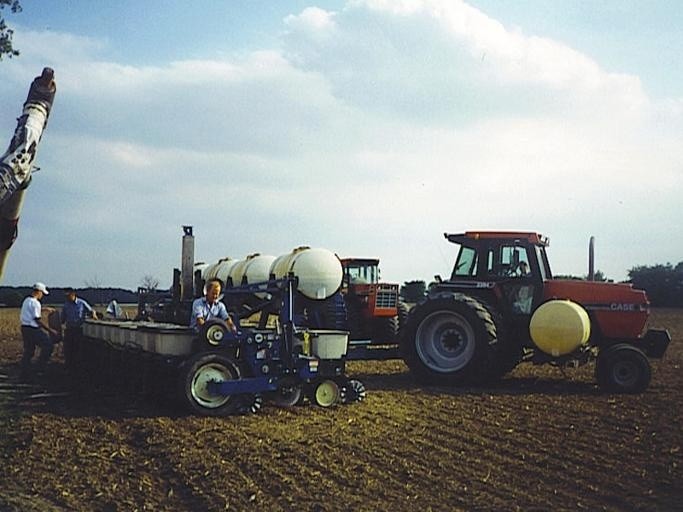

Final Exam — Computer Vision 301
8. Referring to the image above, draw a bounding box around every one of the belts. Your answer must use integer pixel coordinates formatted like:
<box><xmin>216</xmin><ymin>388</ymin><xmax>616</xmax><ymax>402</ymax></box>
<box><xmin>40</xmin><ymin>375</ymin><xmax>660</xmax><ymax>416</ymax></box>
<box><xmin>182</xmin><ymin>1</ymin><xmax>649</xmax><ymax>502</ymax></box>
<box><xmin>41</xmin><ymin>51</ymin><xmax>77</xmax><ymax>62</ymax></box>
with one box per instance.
<box><xmin>21</xmin><ymin>325</ymin><xmax>40</xmax><ymax>329</ymax></box>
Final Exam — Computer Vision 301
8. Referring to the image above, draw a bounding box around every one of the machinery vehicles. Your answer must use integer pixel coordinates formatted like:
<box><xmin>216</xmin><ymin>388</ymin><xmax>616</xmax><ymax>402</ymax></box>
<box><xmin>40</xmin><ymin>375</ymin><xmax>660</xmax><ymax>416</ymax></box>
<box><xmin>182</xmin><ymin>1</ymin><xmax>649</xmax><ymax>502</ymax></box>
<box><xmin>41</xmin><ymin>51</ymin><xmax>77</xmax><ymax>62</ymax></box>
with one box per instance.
<box><xmin>81</xmin><ymin>224</ymin><xmax>410</xmax><ymax>415</ymax></box>
<box><xmin>398</xmin><ymin>229</ymin><xmax>669</xmax><ymax>394</ymax></box>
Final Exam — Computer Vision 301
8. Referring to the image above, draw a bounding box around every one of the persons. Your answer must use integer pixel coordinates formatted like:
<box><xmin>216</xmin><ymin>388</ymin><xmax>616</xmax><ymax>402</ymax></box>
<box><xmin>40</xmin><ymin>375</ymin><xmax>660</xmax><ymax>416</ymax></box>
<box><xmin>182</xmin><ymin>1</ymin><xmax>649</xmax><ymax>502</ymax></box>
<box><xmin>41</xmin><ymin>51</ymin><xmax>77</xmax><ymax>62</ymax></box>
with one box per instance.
<box><xmin>19</xmin><ymin>281</ymin><xmax>58</xmax><ymax>376</ymax></box>
<box><xmin>53</xmin><ymin>289</ymin><xmax>99</xmax><ymax>372</ymax></box>
<box><xmin>189</xmin><ymin>278</ymin><xmax>238</xmax><ymax>357</ymax></box>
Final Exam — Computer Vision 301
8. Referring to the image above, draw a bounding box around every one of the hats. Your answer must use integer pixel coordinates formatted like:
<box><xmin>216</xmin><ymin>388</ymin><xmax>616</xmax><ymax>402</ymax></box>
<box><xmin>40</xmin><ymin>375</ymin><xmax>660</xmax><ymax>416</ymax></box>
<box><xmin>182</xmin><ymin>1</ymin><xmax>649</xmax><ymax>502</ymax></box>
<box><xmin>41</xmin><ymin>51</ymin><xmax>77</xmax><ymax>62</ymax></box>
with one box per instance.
<box><xmin>33</xmin><ymin>283</ymin><xmax>49</xmax><ymax>295</ymax></box>
